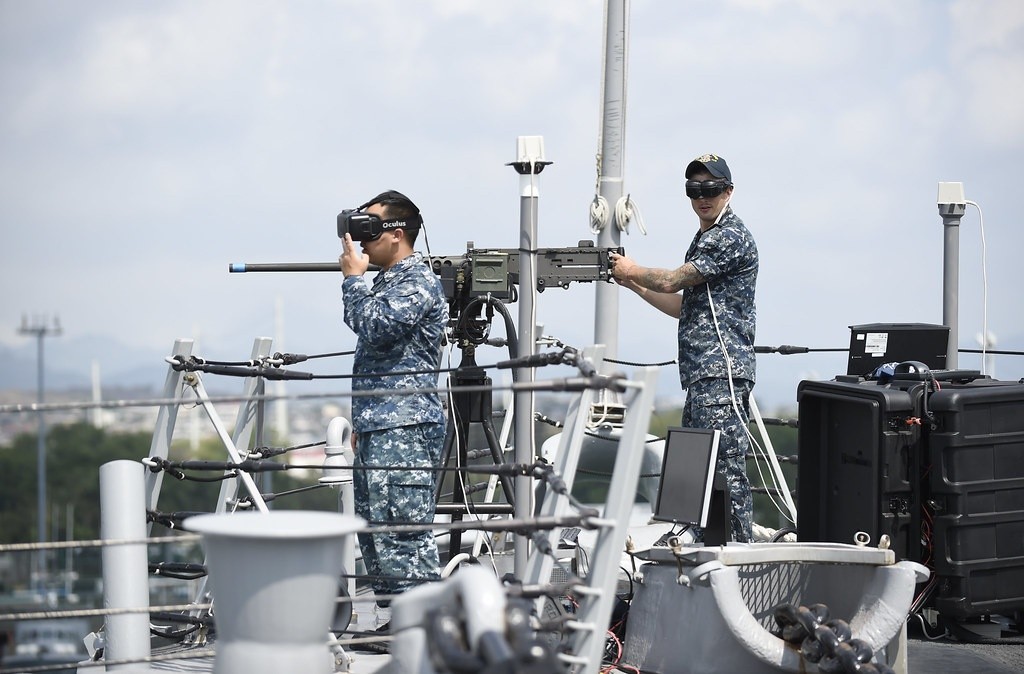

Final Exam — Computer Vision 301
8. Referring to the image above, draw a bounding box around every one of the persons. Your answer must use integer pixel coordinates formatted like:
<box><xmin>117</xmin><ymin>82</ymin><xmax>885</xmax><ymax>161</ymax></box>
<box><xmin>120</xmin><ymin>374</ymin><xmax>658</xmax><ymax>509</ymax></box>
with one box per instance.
<box><xmin>339</xmin><ymin>190</ymin><xmax>448</xmax><ymax>652</ymax></box>
<box><xmin>612</xmin><ymin>153</ymin><xmax>758</xmax><ymax>543</ymax></box>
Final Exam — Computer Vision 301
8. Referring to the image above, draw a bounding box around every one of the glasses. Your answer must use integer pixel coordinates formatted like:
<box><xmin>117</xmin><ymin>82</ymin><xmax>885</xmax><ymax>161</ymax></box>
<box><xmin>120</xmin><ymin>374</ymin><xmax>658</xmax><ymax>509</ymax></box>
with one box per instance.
<box><xmin>686</xmin><ymin>180</ymin><xmax>733</xmax><ymax>200</ymax></box>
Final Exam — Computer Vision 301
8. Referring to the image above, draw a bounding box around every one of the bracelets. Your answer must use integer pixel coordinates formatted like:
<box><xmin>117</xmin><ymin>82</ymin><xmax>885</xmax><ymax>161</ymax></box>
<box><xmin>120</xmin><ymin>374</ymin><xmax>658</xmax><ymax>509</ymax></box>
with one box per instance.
<box><xmin>626</xmin><ymin>264</ymin><xmax>635</xmax><ymax>279</ymax></box>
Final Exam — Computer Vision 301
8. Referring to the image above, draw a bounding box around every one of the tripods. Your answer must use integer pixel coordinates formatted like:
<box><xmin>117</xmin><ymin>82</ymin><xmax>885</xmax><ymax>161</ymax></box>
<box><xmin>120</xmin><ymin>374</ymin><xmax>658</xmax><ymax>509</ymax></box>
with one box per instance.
<box><xmin>432</xmin><ymin>337</ymin><xmax>515</xmax><ymax>568</ymax></box>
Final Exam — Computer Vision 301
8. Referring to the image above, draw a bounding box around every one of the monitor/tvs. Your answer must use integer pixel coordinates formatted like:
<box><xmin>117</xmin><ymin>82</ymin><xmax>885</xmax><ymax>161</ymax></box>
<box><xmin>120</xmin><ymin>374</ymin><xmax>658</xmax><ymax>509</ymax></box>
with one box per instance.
<box><xmin>652</xmin><ymin>426</ymin><xmax>721</xmax><ymax>527</ymax></box>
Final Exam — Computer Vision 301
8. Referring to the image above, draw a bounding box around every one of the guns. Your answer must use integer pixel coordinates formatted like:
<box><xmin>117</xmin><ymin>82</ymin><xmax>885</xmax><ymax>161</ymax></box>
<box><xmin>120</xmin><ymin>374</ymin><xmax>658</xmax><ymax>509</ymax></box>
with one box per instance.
<box><xmin>229</xmin><ymin>240</ymin><xmax>626</xmax><ymax>358</ymax></box>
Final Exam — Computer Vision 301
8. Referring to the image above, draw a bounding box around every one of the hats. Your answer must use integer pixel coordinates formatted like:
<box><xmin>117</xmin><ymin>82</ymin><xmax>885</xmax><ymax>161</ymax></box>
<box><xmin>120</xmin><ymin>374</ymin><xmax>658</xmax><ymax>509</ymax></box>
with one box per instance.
<box><xmin>685</xmin><ymin>154</ymin><xmax>732</xmax><ymax>183</ymax></box>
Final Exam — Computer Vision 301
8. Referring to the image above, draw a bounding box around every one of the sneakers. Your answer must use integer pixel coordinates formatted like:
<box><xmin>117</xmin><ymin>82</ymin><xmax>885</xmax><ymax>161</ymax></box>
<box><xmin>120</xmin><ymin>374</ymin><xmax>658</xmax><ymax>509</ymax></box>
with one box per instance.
<box><xmin>348</xmin><ymin>622</ymin><xmax>392</xmax><ymax>654</ymax></box>
